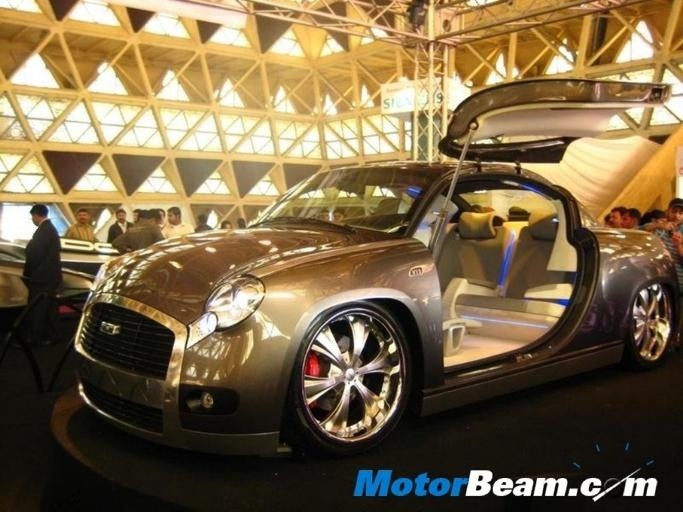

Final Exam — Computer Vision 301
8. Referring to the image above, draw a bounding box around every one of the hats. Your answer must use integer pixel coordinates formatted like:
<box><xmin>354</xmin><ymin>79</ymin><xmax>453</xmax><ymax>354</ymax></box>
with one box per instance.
<box><xmin>670</xmin><ymin>198</ymin><xmax>683</xmax><ymax>208</ymax></box>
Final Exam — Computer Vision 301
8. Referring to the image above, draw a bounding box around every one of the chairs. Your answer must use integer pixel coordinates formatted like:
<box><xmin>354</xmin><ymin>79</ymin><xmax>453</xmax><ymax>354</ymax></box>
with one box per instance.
<box><xmin>436</xmin><ymin>211</ymin><xmax>513</xmax><ymax>293</ymax></box>
<box><xmin>454</xmin><ymin>210</ymin><xmax>577</xmax><ymax>332</ymax></box>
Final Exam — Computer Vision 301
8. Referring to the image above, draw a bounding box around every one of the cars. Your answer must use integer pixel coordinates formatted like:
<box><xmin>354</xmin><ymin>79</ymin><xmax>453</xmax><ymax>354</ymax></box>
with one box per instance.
<box><xmin>0</xmin><ymin>242</ymin><xmax>95</xmax><ymax>351</ymax></box>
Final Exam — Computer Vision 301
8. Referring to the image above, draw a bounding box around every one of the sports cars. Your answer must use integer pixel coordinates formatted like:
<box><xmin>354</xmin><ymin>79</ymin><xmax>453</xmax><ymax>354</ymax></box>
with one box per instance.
<box><xmin>64</xmin><ymin>77</ymin><xmax>681</xmax><ymax>465</ymax></box>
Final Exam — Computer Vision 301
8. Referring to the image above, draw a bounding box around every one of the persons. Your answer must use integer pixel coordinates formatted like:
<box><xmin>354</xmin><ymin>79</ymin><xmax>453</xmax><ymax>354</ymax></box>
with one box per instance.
<box><xmin>108</xmin><ymin>199</ymin><xmax>682</xmax><ymax>349</ymax></box>
<box><xmin>64</xmin><ymin>209</ymin><xmax>100</xmax><ymax>244</ymax></box>
<box><xmin>21</xmin><ymin>202</ymin><xmax>64</xmax><ymax>351</ymax></box>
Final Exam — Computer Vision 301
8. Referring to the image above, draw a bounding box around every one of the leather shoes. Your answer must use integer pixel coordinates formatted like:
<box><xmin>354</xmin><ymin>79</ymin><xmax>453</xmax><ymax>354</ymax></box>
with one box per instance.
<box><xmin>40</xmin><ymin>339</ymin><xmax>63</xmax><ymax>347</ymax></box>
